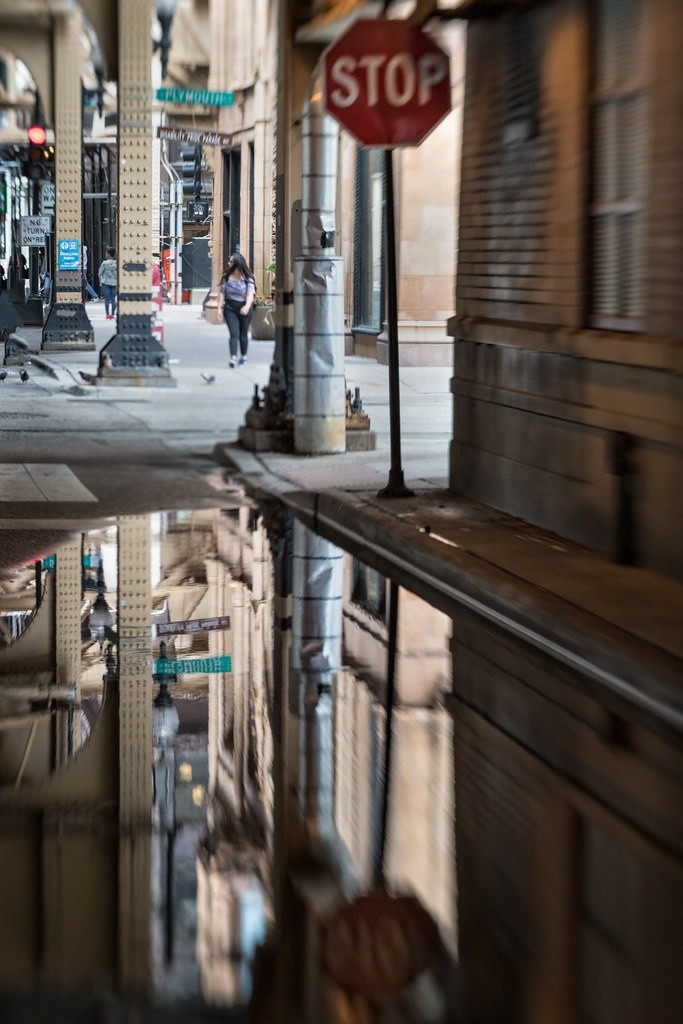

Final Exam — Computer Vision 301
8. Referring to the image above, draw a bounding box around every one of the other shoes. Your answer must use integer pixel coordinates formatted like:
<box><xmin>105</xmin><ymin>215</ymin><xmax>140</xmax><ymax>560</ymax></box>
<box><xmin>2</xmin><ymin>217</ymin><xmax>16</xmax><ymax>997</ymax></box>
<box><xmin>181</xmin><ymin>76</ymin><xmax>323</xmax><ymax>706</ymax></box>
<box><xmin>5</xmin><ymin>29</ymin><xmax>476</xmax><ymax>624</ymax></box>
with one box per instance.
<box><xmin>106</xmin><ymin>315</ymin><xmax>114</xmax><ymax>320</ymax></box>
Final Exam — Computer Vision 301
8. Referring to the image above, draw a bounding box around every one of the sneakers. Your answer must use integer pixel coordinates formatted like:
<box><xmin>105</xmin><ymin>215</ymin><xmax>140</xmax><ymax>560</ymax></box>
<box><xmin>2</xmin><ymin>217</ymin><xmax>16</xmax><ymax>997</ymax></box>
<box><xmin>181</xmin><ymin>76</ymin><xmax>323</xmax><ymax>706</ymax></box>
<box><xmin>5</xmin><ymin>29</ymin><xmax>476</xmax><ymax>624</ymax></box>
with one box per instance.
<box><xmin>88</xmin><ymin>297</ymin><xmax>100</xmax><ymax>304</ymax></box>
<box><xmin>238</xmin><ymin>357</ymin><xmax>247</xmax><ymax>366</ymax></box>
<box><xmin>229</xmin><ymin>360</ymin><xmax>236</xmax><ymax>368</ymax></box>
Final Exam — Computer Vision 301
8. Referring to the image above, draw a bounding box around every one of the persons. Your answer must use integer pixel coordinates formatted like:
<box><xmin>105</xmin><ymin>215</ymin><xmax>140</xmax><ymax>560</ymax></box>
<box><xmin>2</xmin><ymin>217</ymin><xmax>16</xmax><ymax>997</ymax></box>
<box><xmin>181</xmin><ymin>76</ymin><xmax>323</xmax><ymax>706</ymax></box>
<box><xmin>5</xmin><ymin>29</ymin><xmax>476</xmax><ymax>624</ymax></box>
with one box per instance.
<box><xmin>83</xmin><ymin>246</ymin><xmax>100</xmax><ymax>303</ymax></box>
<box><xmin>98</xmin><ymin>247</ymin><xmax>117</xmax><ymax>320</ymax></box>
<box><xmin>39</xmin><ymin>258</ymin><xmax>53</xmax><ymax>306</ymax></box>
<box><xmin>216</xmin><ymin>254</ymin><xmax>256</xmax><ymax>367</ymax></box>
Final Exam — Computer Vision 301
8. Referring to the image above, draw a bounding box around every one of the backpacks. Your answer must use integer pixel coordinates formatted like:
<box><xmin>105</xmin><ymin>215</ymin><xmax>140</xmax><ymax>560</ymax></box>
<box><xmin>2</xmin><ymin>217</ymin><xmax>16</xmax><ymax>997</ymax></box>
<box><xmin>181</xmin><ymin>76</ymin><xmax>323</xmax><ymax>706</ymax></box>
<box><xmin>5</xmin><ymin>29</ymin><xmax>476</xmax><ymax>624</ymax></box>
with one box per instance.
<box><xmin>252</xmin><ymin>300</ymin><xmax>257</xmax><ymax>311</ymax></box>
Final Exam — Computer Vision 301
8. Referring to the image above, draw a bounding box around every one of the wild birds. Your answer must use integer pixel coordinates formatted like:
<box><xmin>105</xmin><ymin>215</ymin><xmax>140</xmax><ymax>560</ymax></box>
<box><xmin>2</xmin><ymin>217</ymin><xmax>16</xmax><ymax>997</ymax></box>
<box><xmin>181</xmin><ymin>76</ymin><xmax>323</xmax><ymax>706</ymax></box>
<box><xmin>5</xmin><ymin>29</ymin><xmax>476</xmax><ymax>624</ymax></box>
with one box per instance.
<box><xmin>21</xmin><ymin>370</ymin><xmax>29</xmax><ymax>383</ymax></box>
<box><xmin>78</xmin><ymin>371</ymin><xmax>98</xmax><ymax>383</ymax></box>
<box><xmin>0</xmin><ymin>372</ymin><xmax>7</xmax><ymax>381</ymax></box>
<box><xmin>201</xmin><ymin>375</ymin><xmax>214</xmax><ymax>383</ymax></box>
